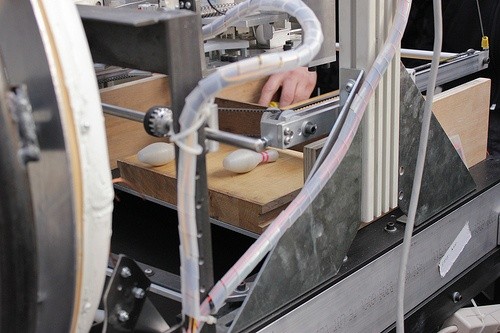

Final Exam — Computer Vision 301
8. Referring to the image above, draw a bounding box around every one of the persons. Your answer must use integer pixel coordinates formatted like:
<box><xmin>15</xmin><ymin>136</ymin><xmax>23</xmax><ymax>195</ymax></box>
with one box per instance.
<box><xmin>255</xmin><ymin>65</ymin><xmax>321</xmax><ymax>109</ymax></box>
